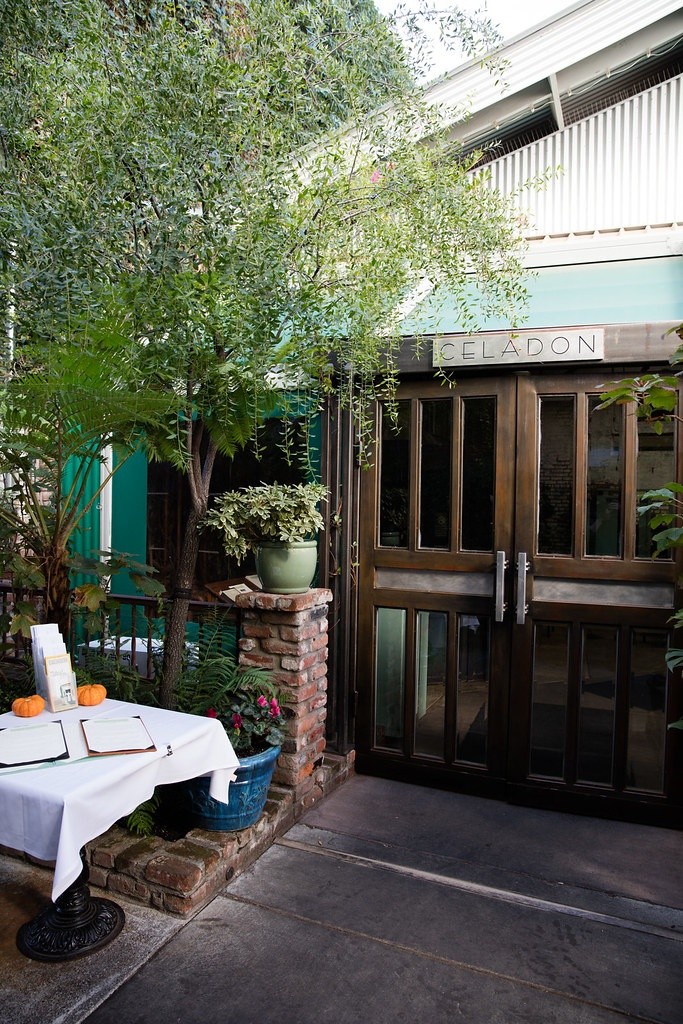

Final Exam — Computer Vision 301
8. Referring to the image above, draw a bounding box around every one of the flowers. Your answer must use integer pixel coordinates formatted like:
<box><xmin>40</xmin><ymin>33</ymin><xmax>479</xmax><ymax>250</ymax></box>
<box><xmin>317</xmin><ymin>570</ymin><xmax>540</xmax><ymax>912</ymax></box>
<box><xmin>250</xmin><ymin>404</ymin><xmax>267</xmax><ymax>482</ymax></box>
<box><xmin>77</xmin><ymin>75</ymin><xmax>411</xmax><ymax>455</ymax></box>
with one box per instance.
<box><xmin>208</xmin><ymin>685</ymin><xmax>292</xmax><ymax>755</ymax></box>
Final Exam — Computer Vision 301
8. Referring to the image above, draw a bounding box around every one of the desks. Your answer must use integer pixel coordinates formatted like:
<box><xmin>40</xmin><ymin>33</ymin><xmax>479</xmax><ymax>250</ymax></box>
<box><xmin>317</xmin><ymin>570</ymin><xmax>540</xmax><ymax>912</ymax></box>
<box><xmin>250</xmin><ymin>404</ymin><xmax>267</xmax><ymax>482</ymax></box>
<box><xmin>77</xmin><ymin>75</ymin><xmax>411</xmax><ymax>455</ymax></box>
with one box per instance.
<box><xmin>0</xmin><ymin>697</ymin><xmax>241</xmax><ymax>965</ymax></box>
<box><xmin>78</xmin><ymin>634</ymin><xmax>200</xmax><ymax>681</ymax></box>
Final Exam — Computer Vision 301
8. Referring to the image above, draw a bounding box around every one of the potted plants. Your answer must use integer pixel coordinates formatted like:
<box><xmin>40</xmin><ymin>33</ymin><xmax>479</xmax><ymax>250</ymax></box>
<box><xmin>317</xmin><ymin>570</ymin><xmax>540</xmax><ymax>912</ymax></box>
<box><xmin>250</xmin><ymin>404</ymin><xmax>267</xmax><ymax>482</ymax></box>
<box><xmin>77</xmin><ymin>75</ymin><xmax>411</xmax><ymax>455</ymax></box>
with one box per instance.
<box><xmin>196</xmin><ymin>479</ymin><xmax>333</xmax><ymax>595</ymax></box>
<box><xmin>381</xmin><ymin>488</ymin><xmax>409</xmax><ymax>547</ymax></box>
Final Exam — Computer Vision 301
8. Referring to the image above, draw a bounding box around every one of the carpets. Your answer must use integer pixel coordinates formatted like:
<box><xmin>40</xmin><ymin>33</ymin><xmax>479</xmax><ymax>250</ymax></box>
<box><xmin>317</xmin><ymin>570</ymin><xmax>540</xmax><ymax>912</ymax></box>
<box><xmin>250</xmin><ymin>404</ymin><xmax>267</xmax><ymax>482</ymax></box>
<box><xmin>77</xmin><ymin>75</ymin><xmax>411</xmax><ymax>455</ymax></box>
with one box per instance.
<box><xmin>462</xmin><ymin>699</ymin><xmax>615</xmax><ymax>782</ymax></box>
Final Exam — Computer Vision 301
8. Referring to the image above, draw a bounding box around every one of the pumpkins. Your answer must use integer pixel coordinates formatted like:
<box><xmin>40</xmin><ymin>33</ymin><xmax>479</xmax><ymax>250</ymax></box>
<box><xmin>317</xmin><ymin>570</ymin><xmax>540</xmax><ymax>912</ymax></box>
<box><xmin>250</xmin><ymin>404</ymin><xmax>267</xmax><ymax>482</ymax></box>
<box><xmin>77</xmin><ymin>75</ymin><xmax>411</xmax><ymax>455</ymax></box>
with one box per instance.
<box><xmin>11</xmin><ymin>695</ymin><xmax>46</xmax><ymax>716</ymax></box>
<box><xmin>77</xmin><ymin>684</ymin><xmax>107</xmax><ymax>706</ymax></box>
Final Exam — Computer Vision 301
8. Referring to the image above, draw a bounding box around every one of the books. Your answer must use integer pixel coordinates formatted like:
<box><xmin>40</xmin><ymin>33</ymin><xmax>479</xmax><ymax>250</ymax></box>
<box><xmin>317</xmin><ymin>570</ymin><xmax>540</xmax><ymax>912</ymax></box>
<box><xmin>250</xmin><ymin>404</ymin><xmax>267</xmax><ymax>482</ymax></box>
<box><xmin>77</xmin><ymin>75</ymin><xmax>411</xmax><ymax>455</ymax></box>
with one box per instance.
<box><xmin>30</xmin><ymin>623</ymin><xmax>78</xmax><ymax>712</ymax></box>
<box><xmin>221</xmin><ymin>588</ymin><xmax>240</xmax><ymax>604</ymax></box>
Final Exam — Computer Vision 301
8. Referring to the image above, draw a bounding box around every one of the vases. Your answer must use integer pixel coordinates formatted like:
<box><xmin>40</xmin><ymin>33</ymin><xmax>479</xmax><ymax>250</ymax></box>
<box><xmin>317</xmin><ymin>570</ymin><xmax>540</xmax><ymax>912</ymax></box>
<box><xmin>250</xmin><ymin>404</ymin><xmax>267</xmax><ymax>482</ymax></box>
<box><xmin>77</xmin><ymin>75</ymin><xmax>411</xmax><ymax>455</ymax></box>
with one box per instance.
<box><xmin>188</xmin><ymin>744</ymin><xmax>288</xmax><ymax>833</ymax></box>
